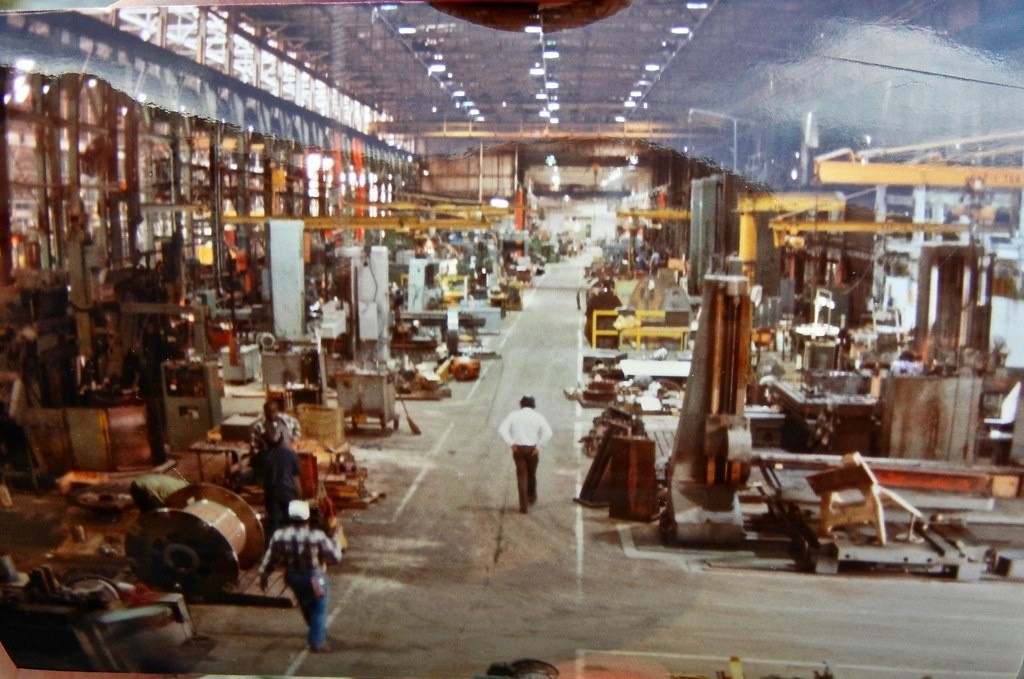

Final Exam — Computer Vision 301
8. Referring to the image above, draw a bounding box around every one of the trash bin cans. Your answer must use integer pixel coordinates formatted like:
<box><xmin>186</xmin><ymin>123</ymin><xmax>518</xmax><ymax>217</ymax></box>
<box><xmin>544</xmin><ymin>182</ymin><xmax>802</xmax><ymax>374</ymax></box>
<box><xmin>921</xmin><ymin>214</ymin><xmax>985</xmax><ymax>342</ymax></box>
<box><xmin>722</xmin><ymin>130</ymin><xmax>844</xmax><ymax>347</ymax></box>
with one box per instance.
<box><xmin>160</xmin><ymin>359</ymin><xmax>223</xmax><ymax>451</ymax></box>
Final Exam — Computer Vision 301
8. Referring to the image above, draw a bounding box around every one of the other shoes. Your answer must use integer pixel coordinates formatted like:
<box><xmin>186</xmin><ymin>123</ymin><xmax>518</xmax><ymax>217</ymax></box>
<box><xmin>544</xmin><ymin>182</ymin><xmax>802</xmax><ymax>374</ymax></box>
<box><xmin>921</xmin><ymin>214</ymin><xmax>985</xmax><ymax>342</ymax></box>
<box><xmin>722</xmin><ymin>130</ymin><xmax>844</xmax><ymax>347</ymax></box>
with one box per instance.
<box><xmin>310</xmin><ymin>640</ymin><xmax>331</xmax><ymax>652</ymax></box>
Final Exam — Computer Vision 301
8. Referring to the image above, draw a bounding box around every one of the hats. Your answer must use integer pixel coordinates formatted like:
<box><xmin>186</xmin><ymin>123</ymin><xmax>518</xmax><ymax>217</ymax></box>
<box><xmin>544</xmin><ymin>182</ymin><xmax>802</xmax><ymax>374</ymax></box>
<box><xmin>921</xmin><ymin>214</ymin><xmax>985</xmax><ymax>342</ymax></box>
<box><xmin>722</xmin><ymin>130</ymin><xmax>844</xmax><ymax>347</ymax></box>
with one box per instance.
<box><xmin>519</xmin><ymin>396</ymin><xmax>536</xmax><ymax>407</ymax></box>
<box><xmin>289</xmin><ymin>500</ymin><xmax>310</xmax><ymax>521</ymax></box>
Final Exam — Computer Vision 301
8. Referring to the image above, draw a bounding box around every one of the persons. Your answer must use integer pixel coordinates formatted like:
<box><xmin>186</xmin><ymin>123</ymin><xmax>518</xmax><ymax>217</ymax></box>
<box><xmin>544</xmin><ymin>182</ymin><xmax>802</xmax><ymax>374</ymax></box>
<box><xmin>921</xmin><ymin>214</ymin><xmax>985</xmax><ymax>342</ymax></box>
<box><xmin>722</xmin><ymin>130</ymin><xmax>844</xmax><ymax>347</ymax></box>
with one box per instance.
<box><xmin>249</xmin><ymin>402</ymin><xmax>301</xmax><ymax>552</ymax></box>
<box><xmin>499</xmin><ymin>395</ymin><xmax>552</xmax><ymax>514</ymax></box>
<box><xmin>649</xmin><ymin>252</ymin><xmax>668</xmax><ymax>274</ymax></box>
<box><xmin>258</xmin><ymin>500</ymin><xmax>341</xmax><ymax>651</ymax></box>
